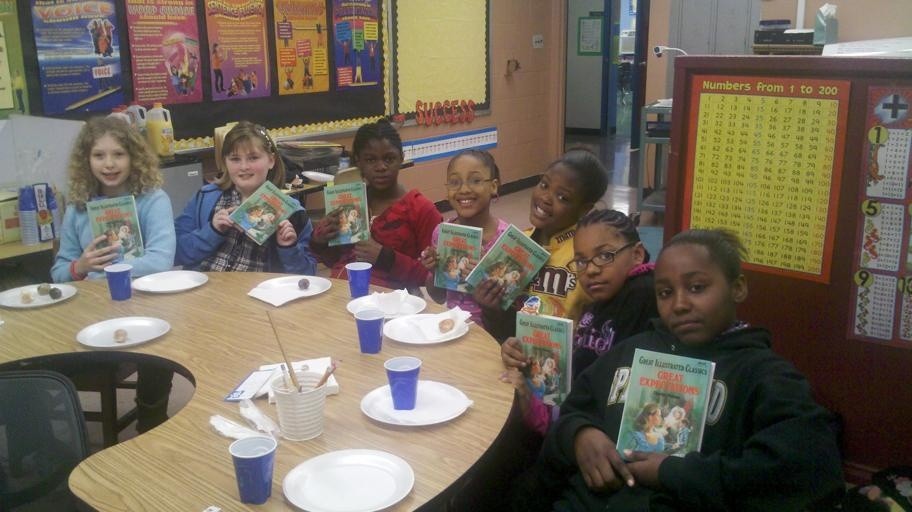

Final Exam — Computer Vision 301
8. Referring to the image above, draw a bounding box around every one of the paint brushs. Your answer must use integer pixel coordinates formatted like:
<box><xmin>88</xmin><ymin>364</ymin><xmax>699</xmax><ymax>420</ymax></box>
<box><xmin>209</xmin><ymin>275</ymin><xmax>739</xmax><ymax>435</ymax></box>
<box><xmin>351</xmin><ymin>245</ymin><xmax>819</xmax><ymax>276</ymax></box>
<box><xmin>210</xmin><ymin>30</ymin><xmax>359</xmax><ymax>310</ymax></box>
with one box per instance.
<box><xmin>267</xmin><ymin>311</ymin><xmax>301</xmax><ymax>392</ymax></box>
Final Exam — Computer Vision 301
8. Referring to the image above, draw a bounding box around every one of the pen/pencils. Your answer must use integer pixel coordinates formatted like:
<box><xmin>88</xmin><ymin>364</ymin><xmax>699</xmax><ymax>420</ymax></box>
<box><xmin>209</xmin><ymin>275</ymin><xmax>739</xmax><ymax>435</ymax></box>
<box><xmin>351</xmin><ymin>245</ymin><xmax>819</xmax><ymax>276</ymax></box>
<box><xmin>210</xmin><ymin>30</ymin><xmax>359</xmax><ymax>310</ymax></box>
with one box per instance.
<box><xmin>281</xmin><ymin>363</ymin><xmax>337</xmax><ymax>388</ymax></box>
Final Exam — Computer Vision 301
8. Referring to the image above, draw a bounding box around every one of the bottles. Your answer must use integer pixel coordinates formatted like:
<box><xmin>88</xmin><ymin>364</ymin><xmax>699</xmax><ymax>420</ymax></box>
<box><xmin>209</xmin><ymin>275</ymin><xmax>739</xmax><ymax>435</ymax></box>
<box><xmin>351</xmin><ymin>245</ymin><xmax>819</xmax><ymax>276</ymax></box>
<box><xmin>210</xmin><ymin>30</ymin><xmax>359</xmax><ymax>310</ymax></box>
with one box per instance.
<box><xmin>339</xmin><ymin>152</ymin><xmax>350</xmax><ymax>169</ymax></box>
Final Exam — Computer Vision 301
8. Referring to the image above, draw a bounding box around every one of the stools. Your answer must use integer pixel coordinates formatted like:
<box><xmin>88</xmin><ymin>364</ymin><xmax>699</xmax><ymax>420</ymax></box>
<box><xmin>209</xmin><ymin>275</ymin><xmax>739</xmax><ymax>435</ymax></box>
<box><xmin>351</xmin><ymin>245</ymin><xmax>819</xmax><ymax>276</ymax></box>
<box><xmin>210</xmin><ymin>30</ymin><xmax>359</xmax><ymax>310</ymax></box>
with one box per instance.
<box><xmin>64</xmin><ymin>357</ymin><xmax>141</xmax><ymax>449</ymax></box>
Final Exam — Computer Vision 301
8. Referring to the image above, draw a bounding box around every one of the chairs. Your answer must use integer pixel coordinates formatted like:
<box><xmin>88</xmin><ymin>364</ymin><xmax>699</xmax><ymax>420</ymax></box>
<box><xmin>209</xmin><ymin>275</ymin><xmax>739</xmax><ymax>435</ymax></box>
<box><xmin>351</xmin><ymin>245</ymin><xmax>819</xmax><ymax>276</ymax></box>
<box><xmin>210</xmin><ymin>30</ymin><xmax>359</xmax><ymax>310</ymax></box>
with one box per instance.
<box><xmin>1</xmin><ymin>367</ymin><xmax>94</xmax><ymax>512</ymax></box>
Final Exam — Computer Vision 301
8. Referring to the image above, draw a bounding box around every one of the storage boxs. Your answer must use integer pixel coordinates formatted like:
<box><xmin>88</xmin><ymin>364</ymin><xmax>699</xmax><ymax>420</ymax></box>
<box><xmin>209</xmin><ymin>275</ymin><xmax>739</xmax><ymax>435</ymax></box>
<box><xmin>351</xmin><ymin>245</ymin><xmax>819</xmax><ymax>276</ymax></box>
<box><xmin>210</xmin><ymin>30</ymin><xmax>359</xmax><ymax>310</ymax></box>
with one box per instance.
<box><xmin>1</xmin><ymin>191</ymin><xmax>23</xmax><ymax>245</ymax></box>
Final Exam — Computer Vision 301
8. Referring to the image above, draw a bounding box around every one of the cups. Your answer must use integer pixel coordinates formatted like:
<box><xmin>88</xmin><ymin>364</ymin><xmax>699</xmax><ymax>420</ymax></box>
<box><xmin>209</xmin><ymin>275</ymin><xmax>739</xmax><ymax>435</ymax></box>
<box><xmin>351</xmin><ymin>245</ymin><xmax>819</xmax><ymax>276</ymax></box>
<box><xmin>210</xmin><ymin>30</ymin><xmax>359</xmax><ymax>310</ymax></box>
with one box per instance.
<box><xmin>17</xmin><ymin>182</ymin><xmax>62</xmax><ymax>245</ymax></box>
<box><xmin>354</xmin><ymin>309</ymin><xmax>384</xmax><ymax>354</ymax></box>
<box><xmin>229</xmin><ymin>436</ymin><xmax>277</xmax><ymax>504</ymax></box>
<box><xmin>383</xmin><ymin>356</ymin><xmax>421</xmax><ymax>412</ymax></box>
<box><xmin>103</xmin><ymin>263</ymin><xmax>132</xmax><ymax>302</ymax></box>
<box><xmin>345</xmin><ymin>261</ymin><xmax>375</xmax><ymax>297</ymax></box>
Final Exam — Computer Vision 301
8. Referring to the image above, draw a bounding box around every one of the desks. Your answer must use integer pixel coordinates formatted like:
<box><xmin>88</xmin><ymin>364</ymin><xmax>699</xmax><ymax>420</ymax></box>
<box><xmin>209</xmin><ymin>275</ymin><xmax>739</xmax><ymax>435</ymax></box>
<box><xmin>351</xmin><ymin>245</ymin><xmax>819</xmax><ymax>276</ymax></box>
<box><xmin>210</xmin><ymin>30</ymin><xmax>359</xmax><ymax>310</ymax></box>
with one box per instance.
<box><xmin>197</xmin><ymin>148</ymin><xmax>416</xmax><ymax>207</ymax></box>
<box><xmin>1</xmin><ymin>268</ymin><xmax>524</xmax><ymax>512</ymax></box>
<box><xmin>1</xmin><ymin>237</ymin><xmax>56</xmax><ymax>283</ymax></box>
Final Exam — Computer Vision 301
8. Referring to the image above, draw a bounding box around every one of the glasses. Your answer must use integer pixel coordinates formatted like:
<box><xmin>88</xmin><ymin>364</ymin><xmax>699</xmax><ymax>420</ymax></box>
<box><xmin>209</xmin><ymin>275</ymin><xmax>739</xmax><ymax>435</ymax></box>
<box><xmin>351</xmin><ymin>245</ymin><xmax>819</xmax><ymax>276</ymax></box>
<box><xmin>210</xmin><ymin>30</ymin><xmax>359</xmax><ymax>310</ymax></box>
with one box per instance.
<box><xmin>566</xmin><ymin>240</ymin><xmax>641</xmax><ymax>276</ymax></box>
<box><xmin>441</xmin><ymin>175</ymin><xmax>498</xmax><ymax>193</ymax></box>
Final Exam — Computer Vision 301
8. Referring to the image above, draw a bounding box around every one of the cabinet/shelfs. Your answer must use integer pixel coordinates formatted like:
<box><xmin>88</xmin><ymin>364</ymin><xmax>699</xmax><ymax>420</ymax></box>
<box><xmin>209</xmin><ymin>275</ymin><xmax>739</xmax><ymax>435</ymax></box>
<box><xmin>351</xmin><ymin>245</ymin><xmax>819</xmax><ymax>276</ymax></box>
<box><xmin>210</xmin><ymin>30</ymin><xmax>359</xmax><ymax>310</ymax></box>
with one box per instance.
<box><xmin>638</xmin><ymin>97</ymin><xmax>675</xmax><ymax>215</ymax></box>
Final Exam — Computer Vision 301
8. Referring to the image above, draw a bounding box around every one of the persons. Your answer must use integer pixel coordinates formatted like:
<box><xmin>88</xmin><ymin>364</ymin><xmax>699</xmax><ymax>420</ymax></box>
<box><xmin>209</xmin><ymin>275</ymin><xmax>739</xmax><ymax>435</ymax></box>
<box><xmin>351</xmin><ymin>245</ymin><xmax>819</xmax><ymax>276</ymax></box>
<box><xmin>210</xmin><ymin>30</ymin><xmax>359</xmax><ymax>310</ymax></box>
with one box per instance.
<box><xmin>336</xmin><ymin>207</ymin><xmax>363</xmax><ymax>244</ymax></box>
<box><xmin>442</xmin><ymin>250</ymin><xmax>476</xmax><ymax>290</ymax></box>
<box><xmin>481</xmin><ymin>262</ymin><xmax>522</xmax><ymax>300</ymax></box>
<box><xmin>337</xmin><ymin>38</ymin><xmax>353</xmax><ymax>66</ymax></box>
<box><xmin>50</xmin><ymin>117</ymin><xmax>177</xmax><ymax>284</ymax></box>
<box><xmin>351</xmin><ymin>49</ymin><xmax>365</xmax><ymax>84</ymax></box>
<box><xmin>625</xmin><ymin>393</ymin><xmax>693</xmax><ymax>455</ymax></box>
<box><xmin>165</xmin><ymin>43</ymin><xmax>260</xmax><ymax>98</ymax></box>
<box><xmin>496</xmin><ymin>208</ymin><xmax>654</xmax><ymax>471</ymax></box>
<box><xmin>88</xmin><ymin>19</ymin><xmax>115</xmax><ymax>58</ymax></box>
<box><xmin>420</xmin><ymin>151</ymin><xmax>509</xmax><ymax>329</ymax></box>
<box><xmin>302</xmin><ymin>55</ymin><xmax>312</xmax><ymax>78</ymax></box>
<box><xmin>104</xmin><ymin>220</ymin><xmax>139</xmax><ymax>264</ymax></box>
<box><xmin>473</xmin><ymin>150</ymin><xmax>608</xmax><ymax>355</ymax></box>
<box><xmin>173</xmin><ymin>121</ymin><xmax>317</xmax><ymax>279</ymax></box>
<box><xmin>316</xmin><ymin>24</ymin><xmax>325</xmax><ymax>49</ymax></box>
<box><xmin>517</xmin><ymin>350</ymin><xmax>561</xmax><ymax>407</ymax></box>
<box><xmin>237</xmin><ymin>203</ymin><xmax>279</xmax><ymax>238</ymax></box>
<box><xmin>310</xmin><ymin>123</ymin><xmax>443</xmax><ymax>299</ymax></box>
<box><xmin>507</xmin><ymin>228</ymin><xmax>848</xmax><ymax>510</ymax></box>
<box><xmin>284</xmin><ymin>63</ymin><xmax>296</xmax><ymax>90</ymax></box>
<box><xmin>364</xmin><ymin>40</ymin><xmax>379</xmax><ymax>68</ymax></box>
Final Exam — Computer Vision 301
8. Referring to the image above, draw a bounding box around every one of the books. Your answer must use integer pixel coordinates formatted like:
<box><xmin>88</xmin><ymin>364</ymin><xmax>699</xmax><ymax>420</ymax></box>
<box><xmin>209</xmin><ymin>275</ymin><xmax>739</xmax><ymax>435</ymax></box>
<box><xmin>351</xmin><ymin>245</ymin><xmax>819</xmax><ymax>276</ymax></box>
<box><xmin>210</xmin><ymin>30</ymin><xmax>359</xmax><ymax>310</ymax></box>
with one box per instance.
<box><xmin>86</xmin><ymin>193</ymin><xmax>145</xmax><ymax>268</ymax></box>
<box><xmin>465</xmin><ymin>223</ymin><xmax>552</xmax><ymax>312</ymax></box>
<box><xmin>275</xmin><ymin>140</ymin><xmax>344</xmax><ymax>176</ymax></box>
<box><xmin>515</xmin><ymin>311</ymin><xmax>574</xmax><ymax>409</ymax></box>
<box><xmin>616</xmin><ymin>344</ymin><xmax>716</xmax><ymax>470</ymax></box>
<box><xmin>226</xmin><ymin>179</ymin><xmax>299</xmax><ymax>246</ymax></box>
<box><xmin>435</xmin><ymin>219</ymin><xmax>481</xmax><ymax>298</ymax></box>
<box><xmin>324</xmin><ymin>179</ymin><xmax>371</xmax><ymax>248</ymax></box>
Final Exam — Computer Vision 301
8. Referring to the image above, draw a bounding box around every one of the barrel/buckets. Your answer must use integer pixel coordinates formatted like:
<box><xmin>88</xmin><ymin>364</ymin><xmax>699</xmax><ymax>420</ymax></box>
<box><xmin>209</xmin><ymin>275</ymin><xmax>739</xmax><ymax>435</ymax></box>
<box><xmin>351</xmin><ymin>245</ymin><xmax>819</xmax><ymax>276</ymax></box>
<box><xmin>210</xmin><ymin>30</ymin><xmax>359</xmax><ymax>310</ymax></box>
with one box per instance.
<box><xmin>127</xmin><ymin>101</ymin><xmax>148</xmax><ymax>145</ymax></box>
<box><xmin>104</xmin><ymin>108</ymin><xmax>132</xmax><ymax>126</ymax></box>
<box><xmin>145</xmin><ymin>102</ymin><xmax>176</xmax><ymax>163</ymax></box>
<box><xmin>117</xmin><ymin>104</ymin><xmax>137</xmax><ymax>131</ymax></box>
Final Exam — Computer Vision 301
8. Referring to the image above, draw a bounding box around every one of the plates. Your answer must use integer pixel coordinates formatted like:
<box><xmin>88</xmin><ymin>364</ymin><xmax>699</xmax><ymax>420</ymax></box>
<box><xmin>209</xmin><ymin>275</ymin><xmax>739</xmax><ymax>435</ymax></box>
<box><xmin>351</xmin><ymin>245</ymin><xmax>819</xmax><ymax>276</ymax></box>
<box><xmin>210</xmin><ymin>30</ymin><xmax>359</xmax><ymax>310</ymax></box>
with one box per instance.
<box><xmin>74</xmin><ymin>314</ymin><xmax>171</xmax><ymax>350</ymax></box>
<box><xmin>384</xmin><ymin>312</ymin><xmax>474</xmax><ymax>345</ymax></box>
<box><xmin>346</xmin><ymin>292</ymin><xmax>428</xmax><ymax>319</ymax></box>
<box><xmin>361</xmin><ymin>381</ymin><xmax>470</xmax><ymax>427</ymax></box>
<box><xmin>301</xmin><ymin>169</ymin><xmax>336</xmax><ymax>183</ymax></box>
<box><xmin>0</xmin><ymin>283</ymin><xmax>77</xmax><ymax>311</ymax></box>
<box><xmin>282</xmin><ymin>449</ymin><xmax>414</xmax><ymax>510</ymax></box>
<box><xmin>255</xmin><ymin>274</ymin><xmax>333</xmax><ymax>297</ymax></box>
<box><xmin>132</xmin><ymin>269</ymin><xmax>206</xmax><ymax>295</ymax></box>
